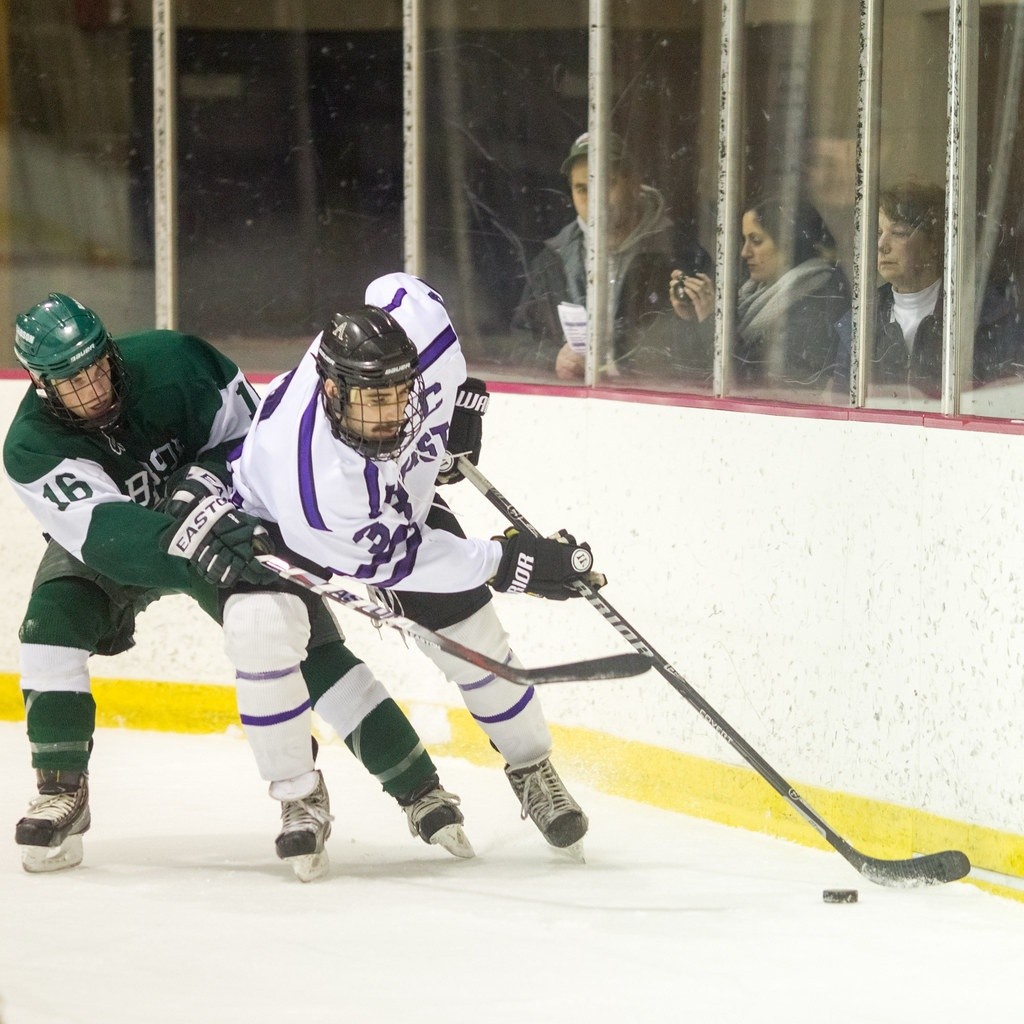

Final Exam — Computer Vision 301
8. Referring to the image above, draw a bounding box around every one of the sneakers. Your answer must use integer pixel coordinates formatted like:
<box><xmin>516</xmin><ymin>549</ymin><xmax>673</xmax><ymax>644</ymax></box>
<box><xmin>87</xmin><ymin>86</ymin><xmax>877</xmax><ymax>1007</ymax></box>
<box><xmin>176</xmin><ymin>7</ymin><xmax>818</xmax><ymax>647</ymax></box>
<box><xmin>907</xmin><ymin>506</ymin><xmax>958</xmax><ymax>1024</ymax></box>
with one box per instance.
<box><xmin>394</xmin><ymin>772</ymin><xmax>476</xmax><ymax>860</ymax></box>
<box><xmin>503</xmin><ymin>748</ymin><xmax>592</xmax><ymax>865</ymax></box>
<box><xmin>14</xmin><ymin>766</ymin><xmax>92</xmax><ymax>873</ymax></box>
<box><xmin>267</xmin><ymin>768</ymin><xmax>335</xmax><ymax>884</ymax></box>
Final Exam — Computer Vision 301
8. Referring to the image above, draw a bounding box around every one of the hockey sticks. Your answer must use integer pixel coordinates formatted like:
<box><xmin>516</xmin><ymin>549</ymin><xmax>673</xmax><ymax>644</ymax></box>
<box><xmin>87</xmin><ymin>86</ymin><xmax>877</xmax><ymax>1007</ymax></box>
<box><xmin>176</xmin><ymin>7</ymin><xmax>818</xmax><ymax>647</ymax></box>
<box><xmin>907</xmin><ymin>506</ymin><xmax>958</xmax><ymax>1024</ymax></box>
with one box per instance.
<box><xmin>257</xmin><ymin>554</ymin><xmax>654</xmax><ymax>687</ymax></box>
<box><xmin>455</xmin><ymin>455</ymin><xmax>971</xmax><ymax>889</ymax></box>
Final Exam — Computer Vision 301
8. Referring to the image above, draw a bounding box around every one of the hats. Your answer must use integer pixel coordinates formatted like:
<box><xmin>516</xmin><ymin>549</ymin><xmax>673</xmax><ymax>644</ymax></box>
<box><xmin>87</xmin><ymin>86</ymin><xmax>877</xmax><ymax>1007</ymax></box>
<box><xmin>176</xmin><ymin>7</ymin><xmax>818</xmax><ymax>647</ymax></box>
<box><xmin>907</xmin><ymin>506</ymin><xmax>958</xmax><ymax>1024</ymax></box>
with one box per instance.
<box><xmin>558</xmin><ymin>129</ymin><xmax>629</xmax><ymax>175</ymax></box>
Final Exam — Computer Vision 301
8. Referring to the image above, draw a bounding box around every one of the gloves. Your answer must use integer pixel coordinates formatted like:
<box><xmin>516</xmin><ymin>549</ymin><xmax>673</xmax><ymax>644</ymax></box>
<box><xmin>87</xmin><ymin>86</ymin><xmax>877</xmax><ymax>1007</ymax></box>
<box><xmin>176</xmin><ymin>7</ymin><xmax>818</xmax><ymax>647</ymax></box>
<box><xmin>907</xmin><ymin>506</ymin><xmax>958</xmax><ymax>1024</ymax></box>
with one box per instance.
<box><xmin>161</xmin><ymin>486</ymin><xmax>277</xmax><ymax>593</ymax></box>
<box><xmin>492</xmin><ymin>529</ymin><xmax>609</xmax><ymax>605</ymax></box>
<box><xmin>434</xmin><ymin>375</ymin><xmax>490</xmax><ymax>487</ymax></box>
<box><xmin>168</xmin><ymin>454</ymin><xmax>239</xmax><ymax>525</ymax></box>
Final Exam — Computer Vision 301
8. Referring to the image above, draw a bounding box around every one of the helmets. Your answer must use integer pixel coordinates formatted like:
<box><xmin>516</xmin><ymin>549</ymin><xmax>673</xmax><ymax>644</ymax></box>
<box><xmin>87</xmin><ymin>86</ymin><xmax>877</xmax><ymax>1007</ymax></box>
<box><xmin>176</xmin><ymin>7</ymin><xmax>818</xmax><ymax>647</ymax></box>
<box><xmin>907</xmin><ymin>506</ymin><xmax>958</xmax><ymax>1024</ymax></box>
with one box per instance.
<box><xmin>309</xmin><ymin>304</ymin><xmax>425</xmax><ymax>461</ymax></box>
<box><xmin>12</xmin><ymin>292</ymin><xmax>133</xmax><ymax>437</ymax></box>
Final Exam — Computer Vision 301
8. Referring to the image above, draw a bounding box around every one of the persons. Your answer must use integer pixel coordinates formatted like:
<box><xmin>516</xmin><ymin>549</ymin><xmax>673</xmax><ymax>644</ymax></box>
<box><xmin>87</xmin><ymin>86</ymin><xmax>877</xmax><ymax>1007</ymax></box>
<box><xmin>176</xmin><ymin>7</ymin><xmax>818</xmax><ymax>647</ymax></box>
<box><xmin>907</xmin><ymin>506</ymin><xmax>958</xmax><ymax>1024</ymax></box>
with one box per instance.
<box><xmin>511</xmin><ymin>129</ymin><xmax>716</xmax><ymax>383</ymax></box>
<box><xmin>219</xmin><ymin>270</ymin><xmax>607</xmax><ymax>860</ymax></box>
<box><xmin>829</xmin><ymin>179</ymin><xmax>1019</xmax><ymax>399</ymax></box>
<box><xmin>668</xmin><ymin>192</ymin><xmax>852</xmax><ymax>389</ymax></box>
<box><xmin>0</xmin><ymin>291</ymin><xmax>463</xmax><ymax>848</ymax></box>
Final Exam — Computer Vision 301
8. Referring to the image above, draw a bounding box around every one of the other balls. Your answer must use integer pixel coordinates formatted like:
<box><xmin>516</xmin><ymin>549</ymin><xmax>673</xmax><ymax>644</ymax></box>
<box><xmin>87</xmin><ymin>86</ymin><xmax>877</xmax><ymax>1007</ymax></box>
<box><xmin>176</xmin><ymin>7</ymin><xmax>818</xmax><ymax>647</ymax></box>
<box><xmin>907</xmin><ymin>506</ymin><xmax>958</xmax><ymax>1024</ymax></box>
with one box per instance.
<box><xmin>823</xmin><ymin>889</ymin><xmax>859</xmax><ymax>904</ymax></box>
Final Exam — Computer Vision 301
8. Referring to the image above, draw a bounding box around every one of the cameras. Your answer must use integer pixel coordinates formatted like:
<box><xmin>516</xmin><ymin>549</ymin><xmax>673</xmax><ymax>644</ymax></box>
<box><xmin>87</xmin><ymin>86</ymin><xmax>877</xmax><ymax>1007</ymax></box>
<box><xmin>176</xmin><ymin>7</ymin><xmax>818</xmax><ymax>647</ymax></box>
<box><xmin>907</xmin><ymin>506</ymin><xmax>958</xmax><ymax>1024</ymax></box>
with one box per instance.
<box><xmin>673</xmin><ymin>270</ymin><xmax>702</xmax><ymax>300</ymax></box>
